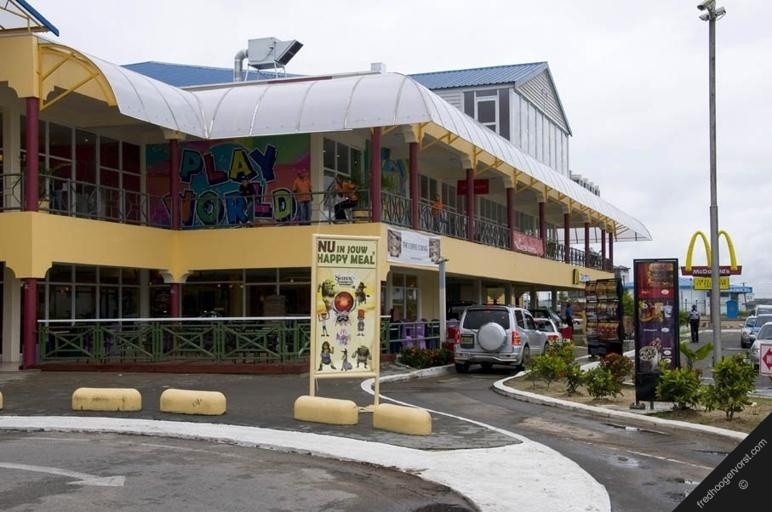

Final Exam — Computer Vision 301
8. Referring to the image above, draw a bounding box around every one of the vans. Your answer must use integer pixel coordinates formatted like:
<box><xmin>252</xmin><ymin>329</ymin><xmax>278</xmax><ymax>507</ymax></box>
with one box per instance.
<box><xmin>534</xmin><ymin>317</ymin><xmax>562</xmax><ymax>355</ymax></box>
<box><xmin>751</xmin><ymin>304</ymin><xmax>772</xmax><ymax>317</ymax></box>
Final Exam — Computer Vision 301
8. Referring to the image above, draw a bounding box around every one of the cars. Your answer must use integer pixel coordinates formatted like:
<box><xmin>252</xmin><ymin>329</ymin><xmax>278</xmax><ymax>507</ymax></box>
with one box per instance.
<box><xmin>749</xmin><ymin>322</ymin><xmax>772</xmax><ymax>366</ymax></box>
<box><xmin>446</xmin><ymin>301</ymin><xmax>478</xmax><ymax>321</ymax></box>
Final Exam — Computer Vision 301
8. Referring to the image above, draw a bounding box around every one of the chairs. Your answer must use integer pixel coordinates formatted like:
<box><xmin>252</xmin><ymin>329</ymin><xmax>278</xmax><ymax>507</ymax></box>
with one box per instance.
<box><xmin>388</xmin><ymin>318</ymin><xmax>439</xmax><ymax>356</ymax></box>
<box><xmin>75</xmin><ymin>323</ymin><xmax>292</xmax><ymax>366</ymax></box>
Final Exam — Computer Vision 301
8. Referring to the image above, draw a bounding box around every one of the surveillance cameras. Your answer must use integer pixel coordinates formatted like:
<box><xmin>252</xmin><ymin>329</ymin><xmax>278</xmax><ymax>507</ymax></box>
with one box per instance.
<box><xmin>696</xmin><ymin>0</ymin><xmax>716</xmax><ymax>11</ymax></box>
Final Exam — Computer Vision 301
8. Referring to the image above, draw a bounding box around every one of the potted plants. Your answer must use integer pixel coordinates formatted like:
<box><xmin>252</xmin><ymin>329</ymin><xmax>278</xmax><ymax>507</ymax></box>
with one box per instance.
<box><xmin>348</xmin><ymin>167</ymin><xmax>393</xmax><ymax>224</ymax></box>
<box><xmin>19</xmin><ymin>156</ymin><xmax>74</xmax><ymax>215</ymax></box>
<box><xmin>620</xmin><ymin>290</ymin><xmax>639</xmax><ymax>340</ymax></box>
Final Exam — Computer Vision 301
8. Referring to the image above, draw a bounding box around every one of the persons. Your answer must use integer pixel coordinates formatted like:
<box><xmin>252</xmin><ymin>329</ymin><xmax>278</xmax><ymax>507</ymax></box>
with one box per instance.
<box><xmin>291</xmin><ymin>167</ymin><xmax>314</xmax><ymax>226</ymax></box>
<box><xmin>332</xmin><ymin>174</ymin><xmax>360</xmax><ymax>224</ymax></box>
<box><xmin>430</xmin><ymin>193</ymin><xmax>443</xmax><ymax>235</ymax></box>
<box><xmin>387</xmin><ymin>316</ymin><xmax>461</xmax><ymax>365</ymax></box>
<box><xmin>565</xmin><ymin>303</ymin><xmax>574</xmax><ymax>334</ymax></box>
<box><xmin>239</xmin><ymin>177</ymin><xmax>257</xmax><ymax>224</ymax></box>
<box><xmin>686</xmin><ymin>304</ymin><xmax>701</xmax><ymax>343</ymax></box>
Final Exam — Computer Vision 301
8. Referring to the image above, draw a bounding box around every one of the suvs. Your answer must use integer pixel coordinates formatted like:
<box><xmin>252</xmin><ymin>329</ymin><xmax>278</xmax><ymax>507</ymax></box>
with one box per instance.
<box><xmin>748</xmin><ymin>314</ymin><xmax>772</xmax><ymax>343</ymax></box>
<box><xmin>739</xmin><ymin>316</ymin><xmax>758</xmax><ymax>347</ymax></box>
<box><xmin>452</xmin><ymin>304</ymin><xmax>550</xmax><ymax>373</ymax></box>
<box><xmin>524</xmin><ymin>306</ymin><xmax>572</xmax><ymax>344</ymax></box>
